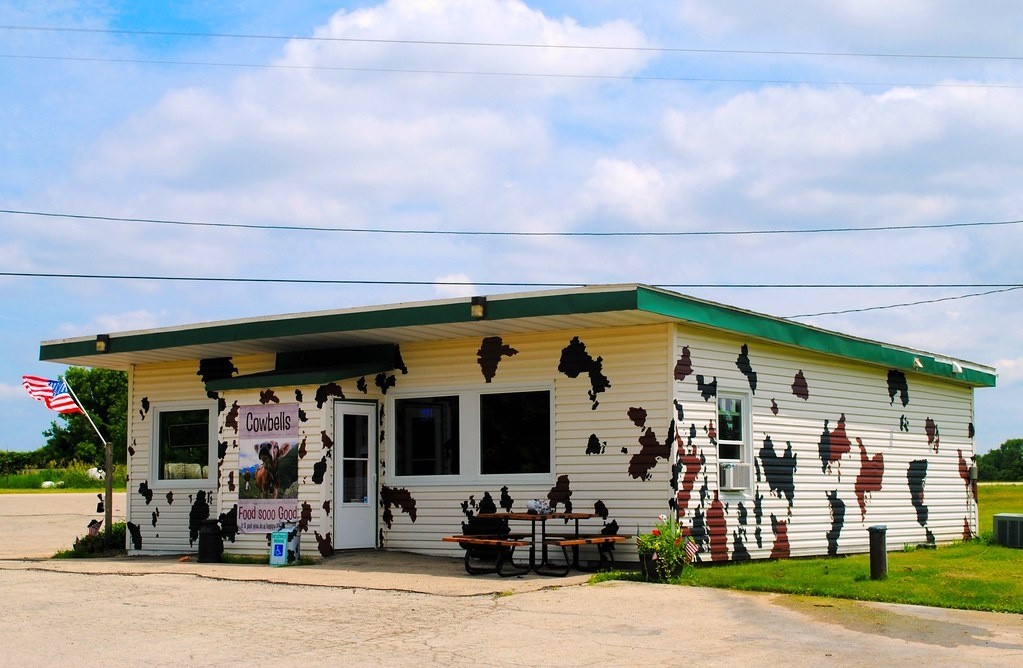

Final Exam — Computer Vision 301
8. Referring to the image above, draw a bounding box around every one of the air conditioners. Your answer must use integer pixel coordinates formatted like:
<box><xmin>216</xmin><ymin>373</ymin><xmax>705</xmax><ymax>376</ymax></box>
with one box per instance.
<box><xmin>721</xmin><ymin>463</ymin><xmax>752</xmax><ymax>492</ymax></box>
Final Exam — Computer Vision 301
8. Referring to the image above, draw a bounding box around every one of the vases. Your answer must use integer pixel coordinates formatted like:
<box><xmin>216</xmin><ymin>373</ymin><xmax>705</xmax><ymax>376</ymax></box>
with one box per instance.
<box><xmin>645</xmin><ymin>552</ymin><xmax>684</xmax><ymax>580</ymax></box>
<box><xmin>639</xmin><ymin>553</ymin><xmax>645</xmax><ymax>576</ymax></box>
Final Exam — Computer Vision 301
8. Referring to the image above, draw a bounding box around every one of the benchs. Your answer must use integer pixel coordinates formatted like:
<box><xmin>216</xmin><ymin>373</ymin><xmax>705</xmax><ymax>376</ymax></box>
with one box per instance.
<box><xmin>442</xmin><ymin>533</ymin><xmax>634</xmax><ymax>578</ymax></box>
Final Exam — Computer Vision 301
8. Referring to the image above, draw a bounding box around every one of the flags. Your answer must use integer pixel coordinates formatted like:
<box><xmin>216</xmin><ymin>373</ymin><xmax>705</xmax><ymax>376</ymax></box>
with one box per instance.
<box><xmin>23</xmin><ymin>376</ymin><xmax>84</xmax><ymax>415</ymax></box>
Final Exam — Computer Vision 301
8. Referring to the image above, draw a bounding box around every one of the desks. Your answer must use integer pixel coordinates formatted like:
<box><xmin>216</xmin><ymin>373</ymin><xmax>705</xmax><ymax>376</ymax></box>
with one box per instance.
<box><xmin>477</xmin><ymin>511</ymin><xmax>599</xmax><ymax>578</ymax></box>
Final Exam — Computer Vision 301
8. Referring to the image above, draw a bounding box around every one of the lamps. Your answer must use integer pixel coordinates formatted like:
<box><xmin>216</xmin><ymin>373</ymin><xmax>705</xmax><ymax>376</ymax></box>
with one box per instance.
<box><xmin>95</xmin><ymin>334</ymin><xmax>110</xmax><ymax>354</ymax></box>
<box><xmin>471</xmin><ymin>295</ymin><xmax>487</xmax><ymax>319</ymax></box>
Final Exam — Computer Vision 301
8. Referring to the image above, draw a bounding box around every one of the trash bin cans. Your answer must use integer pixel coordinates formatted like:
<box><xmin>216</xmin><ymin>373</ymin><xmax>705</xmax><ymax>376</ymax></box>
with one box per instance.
<box><xmin>269</xmin><ymin>521</ymin><xmax>301</xmax><ymax>568</ymax></box>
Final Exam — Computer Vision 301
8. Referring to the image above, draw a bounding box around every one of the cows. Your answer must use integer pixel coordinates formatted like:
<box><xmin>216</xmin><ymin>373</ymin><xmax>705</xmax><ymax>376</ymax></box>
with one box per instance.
<box><xmin>254</xmin><ymin>440</ymin><xmax>290</xmax><ymax>499</ymax></box>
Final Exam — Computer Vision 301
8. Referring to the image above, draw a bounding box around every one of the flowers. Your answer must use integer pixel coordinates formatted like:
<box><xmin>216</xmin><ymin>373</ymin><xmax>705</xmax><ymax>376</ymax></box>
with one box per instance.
<box><xmin>636</xmin><ymin>514</ymin><xmax>693</xmax><ymax>556</ymax></box>
